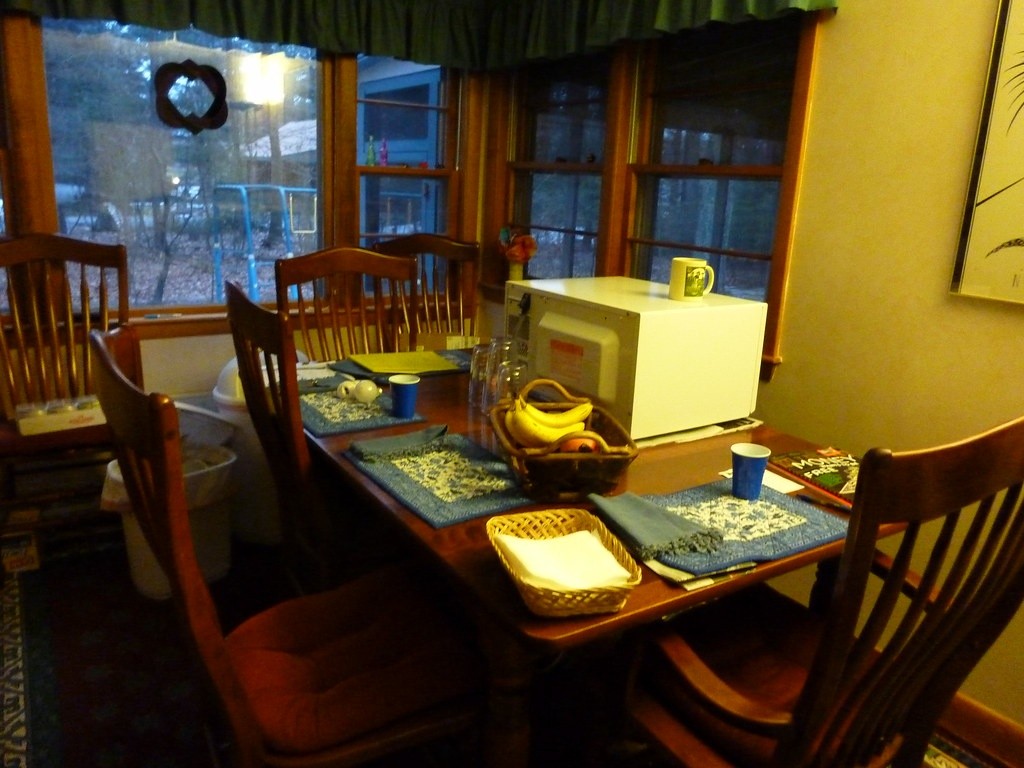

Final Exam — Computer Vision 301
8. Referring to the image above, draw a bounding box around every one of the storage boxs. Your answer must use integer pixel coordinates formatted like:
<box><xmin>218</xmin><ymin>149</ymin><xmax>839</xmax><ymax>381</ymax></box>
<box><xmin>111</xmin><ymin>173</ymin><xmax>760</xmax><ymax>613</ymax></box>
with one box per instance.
<box><xmin>488</xmin><ymin>378</ymin><xmax>639</xmax><ymax>501</ymax></box>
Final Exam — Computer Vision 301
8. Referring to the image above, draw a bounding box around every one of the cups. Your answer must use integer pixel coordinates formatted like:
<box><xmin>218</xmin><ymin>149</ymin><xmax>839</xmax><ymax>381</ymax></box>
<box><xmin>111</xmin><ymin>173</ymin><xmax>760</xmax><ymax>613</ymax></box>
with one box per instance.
<box><xmin>469</xmin><ymin>345</ymin><xmax>491</xmax><ymax>406</ymax></box>
<box><xmin>483</xmin><ymin>339</ymin><xmax>520</xmax><ymax>415</ymax></box>
<box><xmin>731</xmin><ymin>442</ymin><xmax>771</xmax><ymax>500</ymax></box>
<box><xmin>496</xmin><ymin>361</ymin><xmax>527</xmax><ymax>403</ymax></box>
<box><xmin>668</xmin><ymin>256</ymin><xmax>714</xmax><ymax>303</ymax></box>
<box><xmin>389</xmin><ymin>375</ymin><xmax>421</xmax><ymax>419</ymax></box>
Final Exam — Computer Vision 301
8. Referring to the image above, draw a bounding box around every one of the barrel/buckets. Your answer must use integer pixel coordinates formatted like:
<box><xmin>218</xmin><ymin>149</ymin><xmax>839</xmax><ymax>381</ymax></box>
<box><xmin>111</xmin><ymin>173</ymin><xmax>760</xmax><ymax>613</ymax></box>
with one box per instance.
<box><xmin>105</xmin><ymin>448</ymin><xmax>237</xmax><ymax>596</ymax></box>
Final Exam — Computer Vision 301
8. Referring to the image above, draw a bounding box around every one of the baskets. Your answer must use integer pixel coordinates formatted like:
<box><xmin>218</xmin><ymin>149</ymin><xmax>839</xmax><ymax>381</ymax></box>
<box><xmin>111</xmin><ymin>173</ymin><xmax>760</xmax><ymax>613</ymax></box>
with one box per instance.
<box><xmin>488</xmin><ymin>508</ymin><xmax>641</xmax><ymax>618</ymax></box>
<box><xmin>489</xmin><ymin>377</ymin><xmax>639</xmax><ymax>496</ymax></box>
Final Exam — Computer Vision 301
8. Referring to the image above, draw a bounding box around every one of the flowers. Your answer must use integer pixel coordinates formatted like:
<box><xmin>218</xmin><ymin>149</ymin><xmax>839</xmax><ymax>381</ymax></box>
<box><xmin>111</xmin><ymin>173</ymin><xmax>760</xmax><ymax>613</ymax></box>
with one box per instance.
<box><xmin>496</xmin><ymin>226</ymin><xmax>537</xmax><ymax>262</ymax></box>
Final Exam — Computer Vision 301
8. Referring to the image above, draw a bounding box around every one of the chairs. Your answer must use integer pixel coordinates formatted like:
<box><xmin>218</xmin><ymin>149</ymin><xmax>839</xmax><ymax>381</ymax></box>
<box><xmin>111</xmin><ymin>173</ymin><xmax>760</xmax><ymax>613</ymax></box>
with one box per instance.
<box><xmin>372</xmin><ymin>232</ymin><xmax>481</xmax><ymax>352</ymax></box>
<box><xmin>275</xmin><ymin>245</ymin><xmax>419</xmax><ymax>362</ymax></box>
<box><xmin>583</xmin><ymin>421</ymin><xmax>1024</xmax><ymax>768</ymax></box>
<box><xmin>225</xmin><ymin>280</ymin><xmax>403</xmax><ymax>590</ymax></box>
<box><xmin>89</xmin><ymin>322</ymin><xmax>491</xmax><ymax>768</ymax></box>
<box><xmin>0</xmin><ymin>233</ymin><xmax>128</xmax><ymax>584</ymax></box>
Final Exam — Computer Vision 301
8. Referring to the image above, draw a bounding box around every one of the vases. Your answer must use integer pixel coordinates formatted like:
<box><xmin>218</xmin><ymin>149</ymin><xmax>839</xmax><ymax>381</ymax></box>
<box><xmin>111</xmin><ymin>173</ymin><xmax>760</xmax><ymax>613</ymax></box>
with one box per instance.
<box><xmin>509</xmin><ymin>261</ymin><xmax>523</xmax><ymax>282</ymax></box>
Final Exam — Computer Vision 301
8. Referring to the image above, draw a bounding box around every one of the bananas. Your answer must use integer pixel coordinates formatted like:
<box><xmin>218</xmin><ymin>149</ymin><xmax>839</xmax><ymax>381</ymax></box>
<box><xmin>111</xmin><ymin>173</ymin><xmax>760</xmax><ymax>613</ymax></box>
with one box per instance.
<box><xmin>504</xmin><ymin>390</ymin><xmax>593</xmax><ymax>448</ymax></box>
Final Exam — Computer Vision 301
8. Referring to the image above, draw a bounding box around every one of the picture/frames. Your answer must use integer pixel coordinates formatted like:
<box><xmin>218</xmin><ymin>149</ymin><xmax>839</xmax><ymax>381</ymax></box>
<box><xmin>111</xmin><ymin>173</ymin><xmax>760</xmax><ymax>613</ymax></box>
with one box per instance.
<box><xmin>947</xmin><ymin>0</ymin><xmax>1024</xmax><ymax>306</ymax></box>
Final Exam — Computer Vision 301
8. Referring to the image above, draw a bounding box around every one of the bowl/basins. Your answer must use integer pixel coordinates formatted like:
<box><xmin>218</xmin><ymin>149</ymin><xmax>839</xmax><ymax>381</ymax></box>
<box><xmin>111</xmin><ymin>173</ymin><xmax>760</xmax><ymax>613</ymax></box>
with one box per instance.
<box><xmin>170</xmin><ymin>403</ymin><xmax>238</xmax><ymax>445</ymax></box>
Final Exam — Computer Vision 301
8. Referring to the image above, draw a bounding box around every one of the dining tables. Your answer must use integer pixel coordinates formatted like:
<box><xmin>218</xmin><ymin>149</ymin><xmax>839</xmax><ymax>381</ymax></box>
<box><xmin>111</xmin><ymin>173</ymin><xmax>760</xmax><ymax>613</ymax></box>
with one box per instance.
<box><xmin>303</xmin><ymin>342</ymin><xmax>909</xmax><ymax>768</ymax></box>
